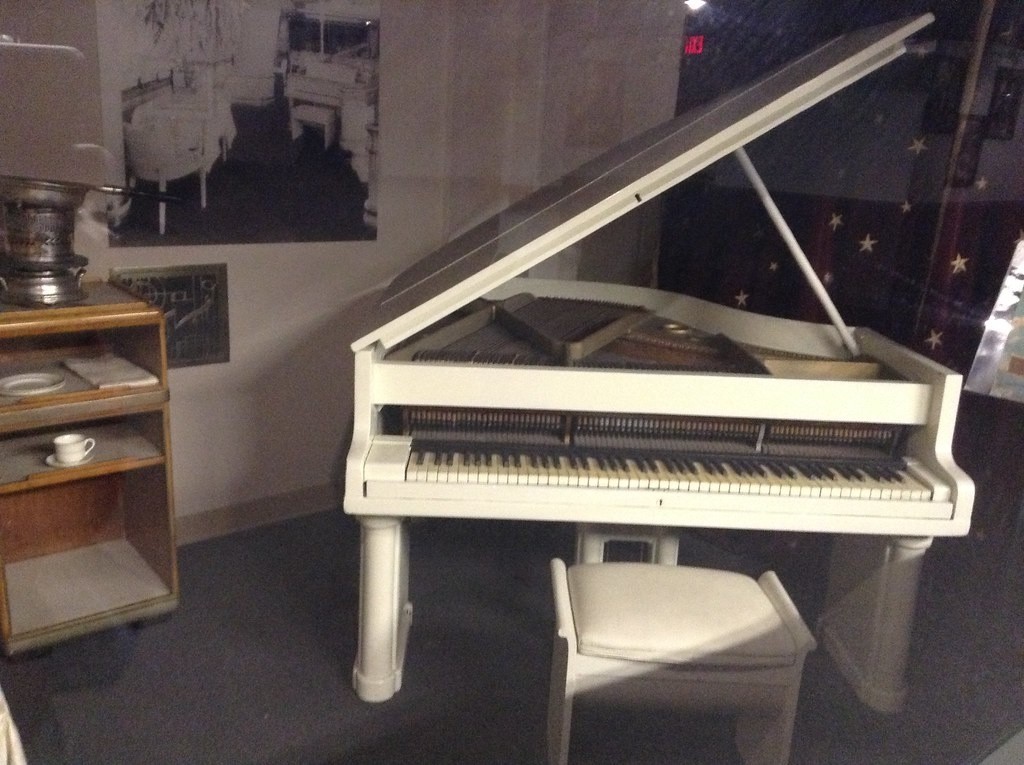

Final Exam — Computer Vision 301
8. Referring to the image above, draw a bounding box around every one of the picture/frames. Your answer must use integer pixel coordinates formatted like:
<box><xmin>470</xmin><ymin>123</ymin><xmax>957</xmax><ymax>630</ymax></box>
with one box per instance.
<box><xmin>110</xmin><ymin>263</ymin><xmax>231</xmax><ymax>369</ymax></box>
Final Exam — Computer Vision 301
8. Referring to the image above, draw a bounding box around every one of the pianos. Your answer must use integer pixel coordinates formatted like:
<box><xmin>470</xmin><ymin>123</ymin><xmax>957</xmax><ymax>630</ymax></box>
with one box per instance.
<box><xmin>343</xmin><ymin>10</ymin><xmax>971</xmax><ymax>716</ymax></box>
<box><xmin>285</xmin><ymin>48</ymin><xmax>379</xmax><ymax>152</ymax></box>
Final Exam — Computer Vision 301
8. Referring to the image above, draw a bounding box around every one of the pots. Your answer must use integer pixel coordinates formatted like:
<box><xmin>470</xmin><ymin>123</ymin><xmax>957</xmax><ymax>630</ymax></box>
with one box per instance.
<box><xmin>0</xmin><ymin>171</ymin><xmax>187</xmax><ymax>309</ymax></box>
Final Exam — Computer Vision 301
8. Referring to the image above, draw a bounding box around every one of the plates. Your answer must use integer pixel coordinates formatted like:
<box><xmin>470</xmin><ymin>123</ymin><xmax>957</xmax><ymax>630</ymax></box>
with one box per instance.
<box><xmin>0</xmin><ymin>372</ymin><xmax>66</xmax><ymax>396</ymax></box>
<box><xmin>46</xmin><ymin>453</ymin><xmax>96</xmax><ymax>467</ymax></box>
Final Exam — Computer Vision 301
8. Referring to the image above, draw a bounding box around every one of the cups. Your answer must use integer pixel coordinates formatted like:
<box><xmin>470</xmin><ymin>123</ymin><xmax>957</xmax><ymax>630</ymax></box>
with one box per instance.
<box><xmin>53</xmin><ymin>434</ymin><xmax>95</xmax><ymax>463</ymax></box>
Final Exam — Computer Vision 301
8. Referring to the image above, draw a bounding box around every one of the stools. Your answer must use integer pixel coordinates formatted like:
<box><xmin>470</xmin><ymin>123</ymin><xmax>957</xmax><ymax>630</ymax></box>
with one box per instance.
<box><xmin>574</xmin><ymin>523</ymin><xmax>681</xmax><ymax>565</ymax></box>
<box><xmin>549</xmin><ymin>558</ymin><xmax>819</xmax><ymax>765</ymax></box>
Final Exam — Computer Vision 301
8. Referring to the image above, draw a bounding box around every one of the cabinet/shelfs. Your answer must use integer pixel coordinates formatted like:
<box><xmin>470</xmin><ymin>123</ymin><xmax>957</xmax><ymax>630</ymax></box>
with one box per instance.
<box><xmin>0</xmin><ymin>280</ymin><xmax>180</xmax><ymax>657</ymax></box>
